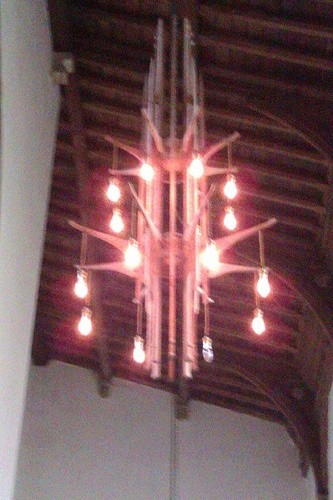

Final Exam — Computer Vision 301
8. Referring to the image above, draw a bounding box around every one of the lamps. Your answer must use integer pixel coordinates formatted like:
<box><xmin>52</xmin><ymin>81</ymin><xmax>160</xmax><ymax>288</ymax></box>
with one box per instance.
<box><xmin>68</xmin><ymin>100</ymin><xmax>284</xmax><ymax>386</ymax></box>
